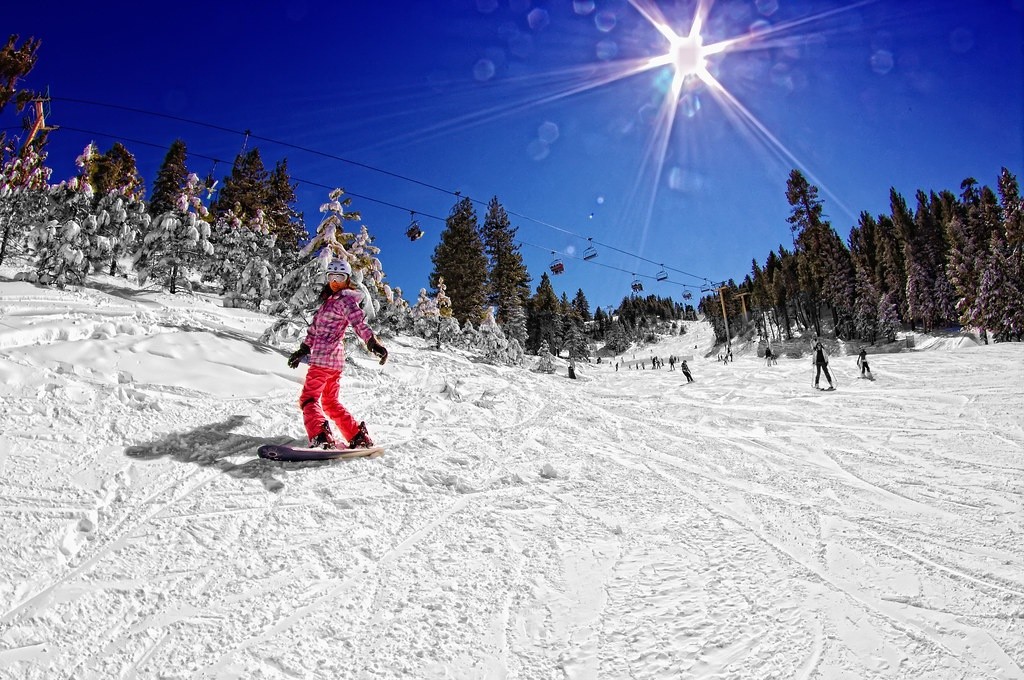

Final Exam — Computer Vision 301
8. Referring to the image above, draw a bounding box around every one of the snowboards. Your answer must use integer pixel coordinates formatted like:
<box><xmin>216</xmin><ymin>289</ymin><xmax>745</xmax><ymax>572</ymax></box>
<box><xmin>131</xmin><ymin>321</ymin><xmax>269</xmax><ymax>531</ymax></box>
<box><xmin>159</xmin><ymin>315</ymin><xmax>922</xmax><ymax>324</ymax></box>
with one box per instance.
<box><xmin>857</xmin><ymin>376</ymin><xmax>876</xmax><ymax>381</ymax></box>
<box><xmin>257</xmin><ymin>445</ymin><xmax>386</xmax><ymax>460</ymax></box>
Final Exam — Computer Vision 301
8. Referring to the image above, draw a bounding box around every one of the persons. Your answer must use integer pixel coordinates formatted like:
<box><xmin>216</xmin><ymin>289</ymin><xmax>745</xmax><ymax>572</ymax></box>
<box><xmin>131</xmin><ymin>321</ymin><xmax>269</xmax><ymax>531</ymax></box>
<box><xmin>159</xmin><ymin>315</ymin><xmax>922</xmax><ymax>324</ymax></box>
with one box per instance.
<box><xmin>287</xmin><ymin>259</ymin><xmax>388</xmax><ymax>451</ymax></box>
<box><xmin>549</xmin><ymin>263</ymin><xmax>564</xmax><ymax>275</ymax></box>
<box><xmin>567</xmin><ymin>365</ymin><xmax>577</xmax><ymax>380</ymax></box>
<box><xmin>857</xmin><ymin>345</ymin><xmax>875</xmax><ymax>381</ymax></box>
<box><xmin>631</xmin><ymin>283</ymin><xmax>644</xmax><ymax>293</ymax></box>
<box><xmin>683</xmin><ymin>293</ymin><xmax>692</xmax><ymax>300</ymax></box>
<box><xmin>694</xmin><ymin>344</ymin><xmax>699</xmax><ymax>349</ymax></box>
<box><xmin>587</xmin><ymin>355</ymin><xmax>694</xmax><ymax>382</ymax></box>
<box><xmin>717</xmin><ymin>341</ymin><xmax>734</xmax><ymax>366</ymax></box>
<box><xmin>764</xmin><ymin>347</ymin><xmax>777</xmax><ymax>366</ymax></box>
<box><xmin>406</xmin><ymin>225</ymin><xmax>418</xmax><ymax>235</ymax></box>
<box><xmin>808</xmin><ymin>333</ymin><xmax>838</xmax><ymax>392</ymax></box>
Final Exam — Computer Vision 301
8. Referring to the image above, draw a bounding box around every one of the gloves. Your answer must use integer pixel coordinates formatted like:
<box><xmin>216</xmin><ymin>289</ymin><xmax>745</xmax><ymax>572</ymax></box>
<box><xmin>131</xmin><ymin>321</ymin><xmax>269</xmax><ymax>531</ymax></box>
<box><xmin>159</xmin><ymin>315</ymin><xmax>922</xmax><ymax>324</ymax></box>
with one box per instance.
<box><xmin>368</xmin><ymin>338</ymin><xmax>388</xmax><ymax>366</ymax></box>
<box><xmin>288</xmin><ymin>342</ymin><xmax>312</xmax><ymax>369</ymax></box>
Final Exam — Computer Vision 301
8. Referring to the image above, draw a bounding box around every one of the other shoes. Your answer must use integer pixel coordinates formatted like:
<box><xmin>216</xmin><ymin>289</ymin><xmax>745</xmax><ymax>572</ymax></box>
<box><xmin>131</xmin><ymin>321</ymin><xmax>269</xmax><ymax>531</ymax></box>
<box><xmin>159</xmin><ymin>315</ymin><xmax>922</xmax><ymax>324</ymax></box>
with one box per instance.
<box><xmin>349</xmin><ymin>421</ymin><xmax>373</xmax><ymax>447</ymax></box>
<box><xmin>310</xmin><ymin>420</ymin><xmax>335</xmax><ymax>450</ymax></box>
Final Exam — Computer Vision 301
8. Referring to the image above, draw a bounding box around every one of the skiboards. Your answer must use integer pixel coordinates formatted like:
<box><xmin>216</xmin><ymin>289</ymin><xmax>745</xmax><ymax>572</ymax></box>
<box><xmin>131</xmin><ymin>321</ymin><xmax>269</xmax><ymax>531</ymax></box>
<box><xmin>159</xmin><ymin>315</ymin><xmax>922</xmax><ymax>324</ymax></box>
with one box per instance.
<box><xmin>811</xmin><ymin>387</ymin><xmax>837</xmax><ymax>391</ymax></box>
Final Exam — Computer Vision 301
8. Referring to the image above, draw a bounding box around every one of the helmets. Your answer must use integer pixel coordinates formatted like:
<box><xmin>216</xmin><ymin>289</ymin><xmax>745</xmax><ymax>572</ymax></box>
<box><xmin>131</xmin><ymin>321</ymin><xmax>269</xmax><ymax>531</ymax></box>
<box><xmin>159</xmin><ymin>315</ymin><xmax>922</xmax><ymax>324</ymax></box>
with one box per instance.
<box><xmin>326</xmin><ymin>259</ymin><xmax>353</xmax><ymax>279</ymax></box>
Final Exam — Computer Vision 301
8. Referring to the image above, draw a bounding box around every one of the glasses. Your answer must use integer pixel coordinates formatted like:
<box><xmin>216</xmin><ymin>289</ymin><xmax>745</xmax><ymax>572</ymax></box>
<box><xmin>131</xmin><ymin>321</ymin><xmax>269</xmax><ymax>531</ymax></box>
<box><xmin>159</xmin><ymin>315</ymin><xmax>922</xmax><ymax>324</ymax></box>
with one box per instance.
<box><xmin>327</xmin><ymin>273</ymin><xmax>348</xmax><ymax>284</ymax></box>
